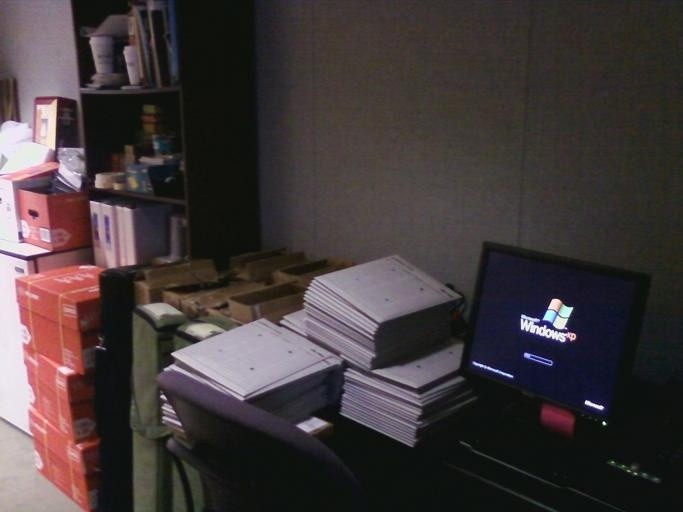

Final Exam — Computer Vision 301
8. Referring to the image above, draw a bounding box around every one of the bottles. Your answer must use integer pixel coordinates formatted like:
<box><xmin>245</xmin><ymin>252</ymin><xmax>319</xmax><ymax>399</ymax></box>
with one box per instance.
<box><xmin>169</xmin><ymin>212</ymin><xmax>187</xmax><ymax>260</ymax></box>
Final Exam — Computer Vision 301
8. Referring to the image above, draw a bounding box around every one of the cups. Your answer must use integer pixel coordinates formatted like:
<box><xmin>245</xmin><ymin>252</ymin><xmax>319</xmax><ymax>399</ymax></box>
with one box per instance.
<box><xmin>122</xmin><ymin>46</ymin><xmax>142</xmax><ymax>86</ymax></box>
<box><xmin>88</xmin><ymin>36</ymin><xmax>116</xmax><ymax>74</ymax></box>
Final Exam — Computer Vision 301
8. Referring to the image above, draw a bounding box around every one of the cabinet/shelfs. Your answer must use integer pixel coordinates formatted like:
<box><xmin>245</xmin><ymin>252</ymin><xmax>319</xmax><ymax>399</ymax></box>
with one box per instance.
<box><xmin>68</xmin><ymin>0</ymin><xmax>260</xmax><ymax>263</ymax></box>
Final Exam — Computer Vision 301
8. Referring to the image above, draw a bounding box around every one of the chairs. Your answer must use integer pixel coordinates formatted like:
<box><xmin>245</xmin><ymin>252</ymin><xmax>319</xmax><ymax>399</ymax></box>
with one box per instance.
<box><xmin>157</xmin><ymin>369</ymin><xmax>371</xmax><ymax>512</ymax></box>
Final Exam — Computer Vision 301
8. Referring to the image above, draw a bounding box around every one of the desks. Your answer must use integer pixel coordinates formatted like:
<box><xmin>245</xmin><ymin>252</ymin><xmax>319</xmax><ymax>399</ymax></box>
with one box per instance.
<box><xmin>302</xmin><ymin>371</ymin><xmax>683</xmax><ymax>511</ymax></box>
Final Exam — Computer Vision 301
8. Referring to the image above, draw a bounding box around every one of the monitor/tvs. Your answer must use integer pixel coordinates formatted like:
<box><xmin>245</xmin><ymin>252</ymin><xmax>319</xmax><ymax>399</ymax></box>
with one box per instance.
<box><xmin>459</xmin><ymin>240</ymin><xmax>650</xmax><ymax>424</ymax></box>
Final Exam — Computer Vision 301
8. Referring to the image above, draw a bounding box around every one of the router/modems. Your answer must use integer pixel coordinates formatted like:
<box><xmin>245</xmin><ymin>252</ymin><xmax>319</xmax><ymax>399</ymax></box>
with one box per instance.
<box><xmin>603</xmin><ymin>453</ymin><xmax>673</xmax><ymax>500</ymax></box>
